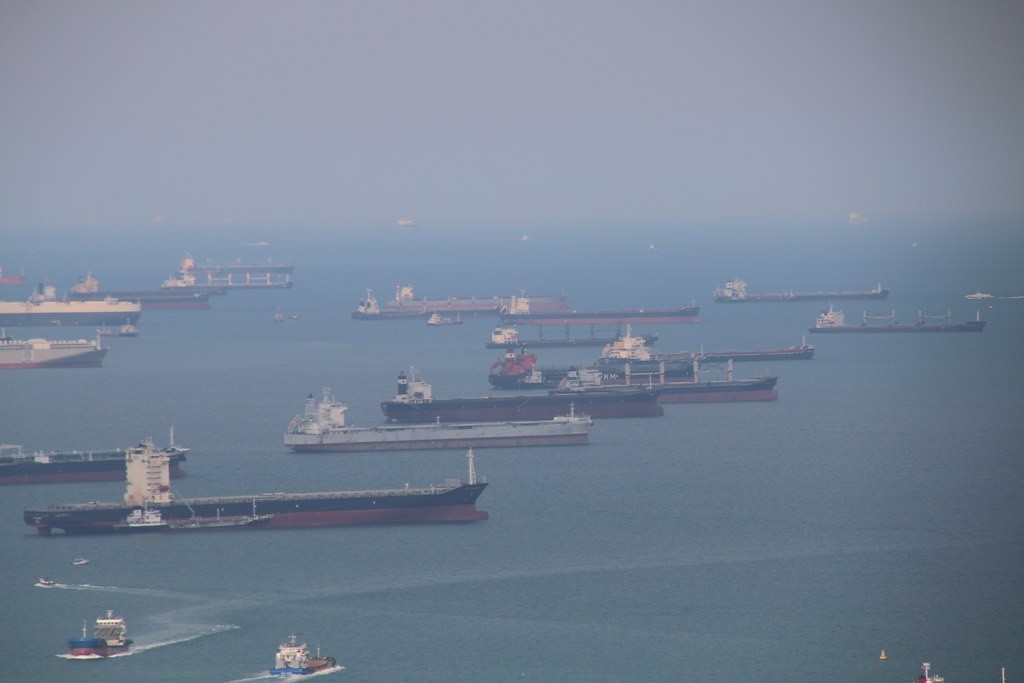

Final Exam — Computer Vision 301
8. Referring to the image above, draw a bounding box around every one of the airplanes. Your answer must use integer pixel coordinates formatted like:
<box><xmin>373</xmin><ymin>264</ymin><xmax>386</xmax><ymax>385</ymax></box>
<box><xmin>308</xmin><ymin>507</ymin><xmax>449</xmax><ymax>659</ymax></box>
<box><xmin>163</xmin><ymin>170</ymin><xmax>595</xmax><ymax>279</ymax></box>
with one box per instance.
<box><xmin>966</xmin><ymin>290</ymin><xmax>1024</xmax><ymax>302</ymax></box>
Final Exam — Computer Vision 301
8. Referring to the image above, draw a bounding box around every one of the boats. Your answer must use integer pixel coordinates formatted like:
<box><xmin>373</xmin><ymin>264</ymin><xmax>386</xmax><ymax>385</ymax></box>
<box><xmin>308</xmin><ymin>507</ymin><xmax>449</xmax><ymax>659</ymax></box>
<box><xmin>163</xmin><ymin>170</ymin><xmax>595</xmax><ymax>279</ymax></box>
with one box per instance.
<box><xmin>157</xmin><ymin>252</ymin><xmax>296</xmax><ymax>290</ymax></box>
<box><xmin>499</xmin><ymin>291</ymin><xmax>699</xmax><ymax>324</ymax></box>
<box><xmin>283</xmin><ymin>384</ymin><xmax>593</xmax><ymax>453</ymax></box>
<box><xmin>709</xmin><ymin>276</ymin><xmax>893</xmax><ymax>303</ymax></box>
<box><xmin>0</xmin><ymin>280</ymin><xmax>140</xmax><ymax>328</ymax></box>
<box><xmin>349</xmin><ymin>283</ymin><xmax>569</xmax><ymax>319</ymax></box>
<box><xmin>484</xmin><ymin>324</ymin><xmax>662</xmax><ymax>349</ymax></box>
<box><xmin>595</xmin><ymin>318</ymin><xmax>818</xmax><ymax>377</ymax></box>
<box><xmin>70</xmin><ymin>557</ymin><xmax>91</xmax><ymax>567</ymax></box>
<box><xmin>64</xmin><ymin>270</ymin><xmax>213</xmax><ymax>309</ymax></box>
<box><xmin>0</xmin><ymin>425</ymin><xmax>190</xmax><ymax>486</ymax></box>
<box><xmin>269</xmin><ymin>634</ymin><xmax>336</xmax><ymax>677</ymax></box>
<box><xmin>39</xmin><ymin>575</ymin><xmax>54</xmax><ymax>586</ymax></box>
<box><xmin>426</xmin><ymin>309</ymin><xmax>463</xmax><ymax>327</ymax></box>
<box><xmin>24</xmin><ymin>435</ymin><xmax>494</xmax><ymax>536</ymax></box>
<box><xmin>807</xmin><ymin>302</ymin><xmax>990</xmax><ymax>334</ymax></box>
<box><xmin>0</xmin><ymin>330</ymin><xmax>110</xmax><ymax>369</ymax></box>
<box><xmin>96</xmin><ymin>320</ymin><xmax>139</xmax><ymax>339</ymax></box>
<box><xmin>487</xmin><ymin>344</ymin><xmax>780</xmax><ymax>404</ymax></box>
<box><xmin>380</xmin><ymin>364</ymin><xmax>664</xmax><ymax>431</ymax></box>
<box><xmin>68</xmin><ymin>608</ymin><xmax>134</xmax><ymax>657</ymax></box>
